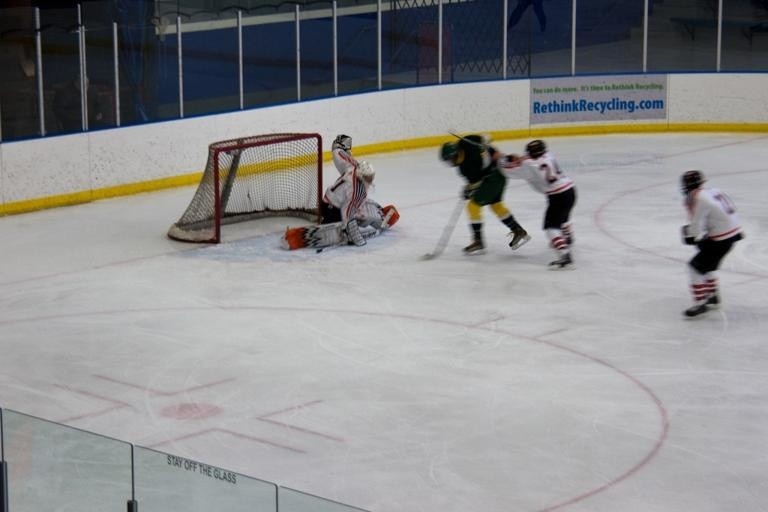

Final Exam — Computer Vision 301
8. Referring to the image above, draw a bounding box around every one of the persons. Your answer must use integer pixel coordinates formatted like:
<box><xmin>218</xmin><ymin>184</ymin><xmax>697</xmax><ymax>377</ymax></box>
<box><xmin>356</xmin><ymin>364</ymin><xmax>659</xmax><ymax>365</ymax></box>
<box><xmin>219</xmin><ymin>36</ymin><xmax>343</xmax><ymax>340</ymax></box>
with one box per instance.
<box><xmin>274</xmin><ymin>134</ymin><xmax>400</xmax><ymax>250</ymax></box>
<box><xmin>493</xmin><ymin>141</ymin><xmax>579</xmax><ymax>266</ymax></box>
<box><xmin>676</xmin><ymin>170</ymin><xmax>743</xmax><ymax>319</ymax></box>
<box><xmin>438</xmin><ymin>132</ymin><xmax>528</xmax><ymax>255</ymax></box>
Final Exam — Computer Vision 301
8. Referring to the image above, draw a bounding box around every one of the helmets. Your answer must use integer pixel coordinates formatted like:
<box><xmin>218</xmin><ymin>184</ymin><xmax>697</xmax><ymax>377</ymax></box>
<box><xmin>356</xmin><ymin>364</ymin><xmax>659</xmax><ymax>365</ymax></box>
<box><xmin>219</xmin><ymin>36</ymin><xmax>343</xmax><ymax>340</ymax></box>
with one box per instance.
<box><xmin>682</xmin><ymin>170</ymin><xmax>702</xmax><ymax>194</ymax></box>
<box><xmin>441</xmin><ymin>141</ymin><xmax>460</xmax><ymax>164</ymax></box>
<box><xmin>354</xmin><ymin>160</ymin><xmax>374</xmax><ymax>188</ymax></box>
<box><xmin>524</xmin><ymin>139</ymin><xmax>547</xmax><ymax>158</ymax></box>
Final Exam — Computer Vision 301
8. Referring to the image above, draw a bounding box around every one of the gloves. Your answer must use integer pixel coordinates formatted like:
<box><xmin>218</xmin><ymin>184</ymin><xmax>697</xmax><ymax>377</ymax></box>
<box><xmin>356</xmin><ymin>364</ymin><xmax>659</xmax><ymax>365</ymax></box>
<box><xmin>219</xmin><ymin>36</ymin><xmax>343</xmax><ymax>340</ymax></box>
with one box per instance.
<box><xmin>463</xmin><ymin>184</ymin><xmax>476</xmax><ymax>200</ymax></box>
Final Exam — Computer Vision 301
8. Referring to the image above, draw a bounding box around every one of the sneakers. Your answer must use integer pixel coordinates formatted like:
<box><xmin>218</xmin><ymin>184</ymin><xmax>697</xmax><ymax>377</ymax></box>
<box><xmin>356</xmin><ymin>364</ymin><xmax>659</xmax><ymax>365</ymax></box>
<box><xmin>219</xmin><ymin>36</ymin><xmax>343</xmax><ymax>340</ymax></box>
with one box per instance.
<box><xmin>510</xmin><ymin>226</ymin><xmax>527</xmax><ymax>247</ymax></box>
<box><xmin>683</xmin><ymin>295</ymin><xmax>720</xmax><ymax>317</ymax></box>
<box><xmin>550</xmin><ymin>252</ymin><xmax>572</xmax><ymax>266</ymax></box>
<box><xmin>463</xmin><ymin>232</ymin><xmax>484</xmax><ymax>253</ymax></box>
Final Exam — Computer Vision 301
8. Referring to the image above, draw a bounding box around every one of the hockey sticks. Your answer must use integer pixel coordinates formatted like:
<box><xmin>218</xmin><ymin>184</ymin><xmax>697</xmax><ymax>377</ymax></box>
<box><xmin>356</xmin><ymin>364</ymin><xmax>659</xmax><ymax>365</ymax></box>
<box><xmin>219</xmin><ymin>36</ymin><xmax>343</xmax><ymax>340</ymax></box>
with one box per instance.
<box><xmin>416</xmin><ymin>199</ymin><xmax>465</xmax><ymax>260</ymax></box>
<box><xmin>448</xmin><ymin>131</ymin><xmax>487</xmax><ymax>147</ymax></box>
